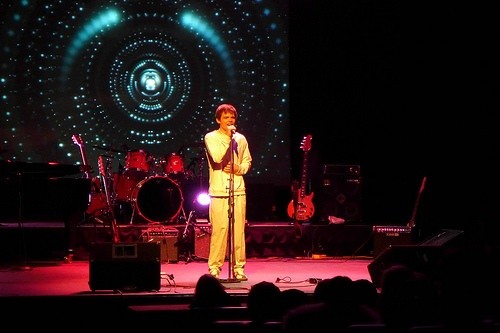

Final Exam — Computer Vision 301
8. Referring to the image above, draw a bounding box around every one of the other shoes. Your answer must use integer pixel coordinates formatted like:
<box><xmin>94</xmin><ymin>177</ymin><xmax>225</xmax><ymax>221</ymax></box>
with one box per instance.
<box><xmin>232</xmin><ymin>267</ymin><xmax>248</xmax><ymax>281</ymax></box>
<box><xmin>211</xmin><ymin>269</ymin><xmax>220</xmax><ymax>280</ymax></box>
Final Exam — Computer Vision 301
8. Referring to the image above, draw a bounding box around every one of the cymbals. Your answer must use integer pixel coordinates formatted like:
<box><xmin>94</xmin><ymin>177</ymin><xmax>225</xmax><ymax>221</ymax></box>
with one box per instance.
<box><xmin>92</xmin><ymin>144</ymin><xmax>124</xmax><ymax>154</ymax></box>
<box><xmin>185</xmin><ymin>141</ymin><xmax>206</xmax><ymax>148</ymax></box>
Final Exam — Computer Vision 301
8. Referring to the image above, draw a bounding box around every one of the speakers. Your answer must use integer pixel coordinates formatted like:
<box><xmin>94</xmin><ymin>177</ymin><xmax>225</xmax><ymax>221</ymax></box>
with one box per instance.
<box><xmin>369</xmin><ymin>243</ymin><xmax>463</xmax><ymax>290</ymax></box>
<box><xmin>421</xmin><ymin>228</ymin><xmax>465</xmax><ymax>247</ymax></box>
<box><xmin>140</xmin><ymin>228</ymin><xmax>178</xmax><ymax>262</ymax></box>
<box><xmin>372</xmin><ymin>225</ymin><xmax>410</xmax><ymax>257</ymax></box>
<box><xmin>191</xmin><ymin>226</ymin><xmax>230</xmax><ymax>260</ymax></box>
<box><xmin>89</xmin><ymin>240</ymin><xmax>161</xmax><ymax>292</ymax></box>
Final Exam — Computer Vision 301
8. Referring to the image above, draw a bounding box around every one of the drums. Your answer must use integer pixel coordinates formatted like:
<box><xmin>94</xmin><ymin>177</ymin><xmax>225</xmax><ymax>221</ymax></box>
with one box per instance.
<box><xmin>128</xmin><ymin>175</ymin><xmax>184</xmax><ymax>224</ymax></box>
<box><xmin>113</xmin><ymin>169</ymin><xmax>138</xmax><ymax>200</ymax></box>
<box><xmin>166</xmin><ymin>153</ymin><xmax>186</xmax><ymax>175</ymax></box>
<box><xmin>124</xmin><ymin>147</ymin><xmax>149</xmax><ymax>174</ymax></box>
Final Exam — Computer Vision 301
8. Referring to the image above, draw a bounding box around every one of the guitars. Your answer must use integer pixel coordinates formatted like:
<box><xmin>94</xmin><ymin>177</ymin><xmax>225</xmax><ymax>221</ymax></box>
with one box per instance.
<box><xmin>71</xmin><ymin>134</ymin><xmax>110</xmax><ymax>216</ymax></box>
<box><xmin>287</xmin><ymin>135</ymin><xmax>316</xmax><ymax>223</ymax></box>
<box><xmin>96</xmin><ymin>156</ymin><xmax>120</xmax><ymax>242</ymax></box>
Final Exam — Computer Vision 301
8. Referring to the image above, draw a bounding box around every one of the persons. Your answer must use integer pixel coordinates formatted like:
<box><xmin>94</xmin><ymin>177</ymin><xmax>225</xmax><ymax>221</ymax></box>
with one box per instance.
<box><xmin>190</xmin><ymin>264</ymin><xmax>437</xmax><ymax>333</ymax></box>
<box><xmin>204</xmin><ymin>104</ymin><xmax>252</xmax><ymax>281</ymax></box>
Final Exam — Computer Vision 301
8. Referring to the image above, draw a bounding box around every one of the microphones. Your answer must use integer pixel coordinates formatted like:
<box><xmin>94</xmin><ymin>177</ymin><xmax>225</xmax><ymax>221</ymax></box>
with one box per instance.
<box><xmin>228</xmin><ymin>123</ymin><xmax>236</xmax><ymax>134</ymax></box>
